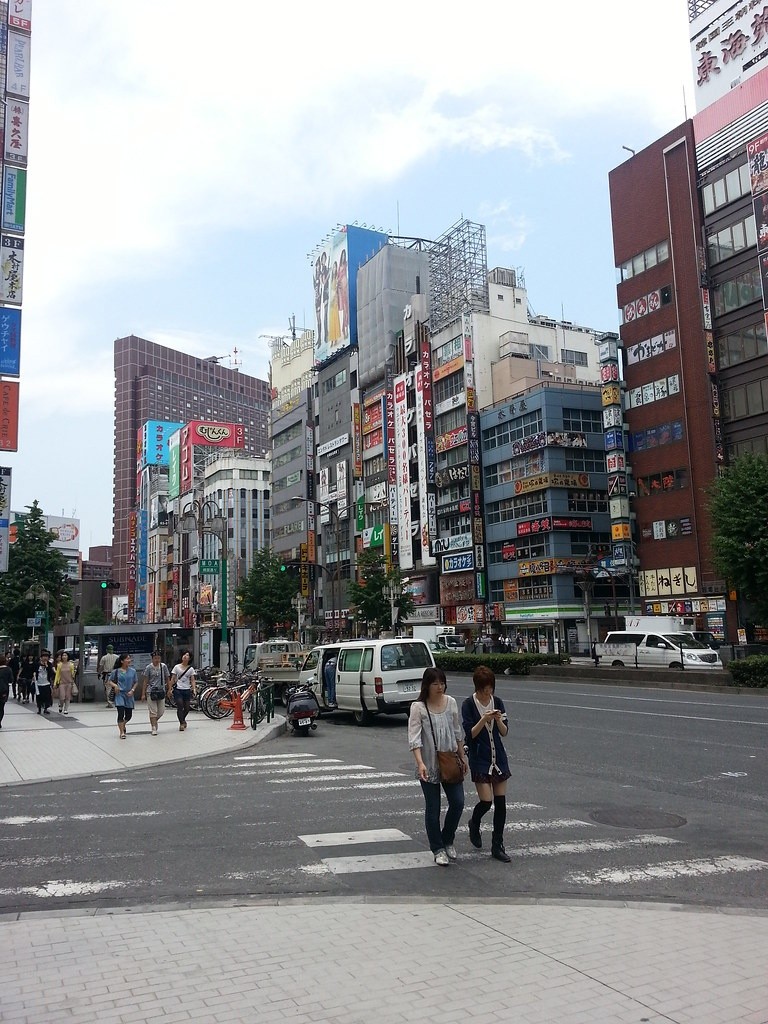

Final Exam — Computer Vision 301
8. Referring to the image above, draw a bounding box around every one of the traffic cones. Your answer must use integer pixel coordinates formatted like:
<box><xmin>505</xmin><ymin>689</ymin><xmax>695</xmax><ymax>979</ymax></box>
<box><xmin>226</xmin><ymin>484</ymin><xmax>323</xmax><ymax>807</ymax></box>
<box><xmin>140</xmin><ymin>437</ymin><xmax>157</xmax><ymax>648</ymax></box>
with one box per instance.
<box><xmin>226</xmin><ymin>693</ymin><xmax>249</xmax><ymax>730</ymax></box>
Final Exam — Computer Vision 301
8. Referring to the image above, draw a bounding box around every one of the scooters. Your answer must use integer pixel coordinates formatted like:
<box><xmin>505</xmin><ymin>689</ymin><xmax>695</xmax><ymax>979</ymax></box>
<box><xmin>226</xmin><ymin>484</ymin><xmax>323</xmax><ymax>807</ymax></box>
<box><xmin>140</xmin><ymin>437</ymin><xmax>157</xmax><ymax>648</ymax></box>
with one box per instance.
<box><xmin>286</xmin><ymin>677</ymin><xmax>319</xmax><ymax>736</ymax></box>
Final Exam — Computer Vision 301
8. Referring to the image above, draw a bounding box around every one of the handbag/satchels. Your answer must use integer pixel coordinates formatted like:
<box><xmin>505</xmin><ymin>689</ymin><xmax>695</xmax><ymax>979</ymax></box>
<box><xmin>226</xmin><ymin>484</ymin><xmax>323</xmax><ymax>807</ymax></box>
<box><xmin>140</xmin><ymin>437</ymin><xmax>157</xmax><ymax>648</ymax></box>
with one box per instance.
<box><xmin>72</xmin><ymin>680</ymin><xmax>78</xmax><ymax>696</ymax></box>
<box><xmin>150</xmin><ymin>687</ymin><xmax>165</xmax><ymax>701</ymax></box>
<box><xmin>30</xmin><ymin>682</ymin><xmax>36</xmax><ymax>694</ymax></box>
<box><xmin>108</xmin><ymin>670</ymin><xmax>118</xmax><ymax>701</ymax></box>
<box><xmin>436</xmin><ymin>751</ymin><xmax>464</xmax><ymax>784</ymax></box>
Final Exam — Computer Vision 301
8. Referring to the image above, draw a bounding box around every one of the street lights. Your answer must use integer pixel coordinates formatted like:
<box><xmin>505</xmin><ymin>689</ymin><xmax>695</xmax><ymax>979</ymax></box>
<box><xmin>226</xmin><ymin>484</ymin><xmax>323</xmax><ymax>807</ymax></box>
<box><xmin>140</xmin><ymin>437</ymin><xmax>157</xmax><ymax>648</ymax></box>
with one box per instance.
<box><xmin>25</xmin><ymin>584</ymin><xmax>47</xmax><ymax>641</ymax></box>
<box><xmin>577</xmin><ymin>582</ymin><xmax>598</xmax><ymax>659</ymax></box>
<box><xmin>127</xmin><ymin>561</ymin><xmax>184</xmax><ymax>623</ymax></box>
<box><xmin>382</xmin><ymin>580</ymin><xmax>403</xmax><ymax>639</ymax></box>
<box><xmin>292</xmin><ymin>497</ymin><xmax>382</xmax><ymax>642</ymax></box>
<box><xmin>176</xmin><ymin>500</ymin><xmax>226</xmax><ymax>626</ymax></box>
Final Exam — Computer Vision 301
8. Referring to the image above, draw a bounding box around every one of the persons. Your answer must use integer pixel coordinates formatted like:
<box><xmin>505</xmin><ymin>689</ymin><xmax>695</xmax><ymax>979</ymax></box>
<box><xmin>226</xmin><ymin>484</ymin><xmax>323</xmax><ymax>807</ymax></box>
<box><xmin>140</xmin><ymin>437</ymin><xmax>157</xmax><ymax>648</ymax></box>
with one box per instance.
<box><xmin>592</xmin><ymin>638</ymin><xmax>600</xmax><ymax>663</ymax></box>
<box><xmin>408</xmin><ymin>668</ymin><xmax>469</xmax><ymax>865</ymax></box>
<box><xmin>141</xmin><ymin>652</ymin><xmax>171</xmax><ymax>735</ymax></box>
<box><xmin>324</xmin><ymin>657</ymin><xmax>338</xmax><ymax>707</ymax></box>
<box><xmin>97</xmin><ymin>645</ymin><xmax>119</xmax><ymax>707</ymax></box>
<box><xmin>461</xmin><ymin>666</ymin><xmax>512</xmax><ymax>862</ymax></box>
<box><xmin>463</xmin><ymin>632</ymin><xmax>536</xmax><ymax>653</ymax></box>
<box><xmin>170</xmin><ymin>650</ymin><xmax>197</xmax><ymax>730</ymax></box>
<box><xmin>108</xmin><ymin>653</ymin><xmax>138</xmax><ymax>738</ymax></box>
<box><xmin>0</xmin><ymin>647</ymin><xmax>75</xmax><ymax>729</ymax></box>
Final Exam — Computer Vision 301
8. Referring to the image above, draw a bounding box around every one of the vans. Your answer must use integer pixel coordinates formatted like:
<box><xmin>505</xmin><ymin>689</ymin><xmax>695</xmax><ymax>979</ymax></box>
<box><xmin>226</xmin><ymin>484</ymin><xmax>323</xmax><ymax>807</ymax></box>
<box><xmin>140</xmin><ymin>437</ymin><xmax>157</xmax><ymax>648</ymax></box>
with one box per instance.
<box><xmin>601</xmin><ymin>630</ymin><xmax>724</xmax><ymax>670</ymax></box>
<box><xmin>296</xmin><ymin>639</ymin><xmax>437</xmax><ymax>726</ymax></box>
<box><xmin>56</xmin><ymin>648</ymin><xmax>80</xmax><ymax>660</ymax></box>
<box><xmin>394</xmin><ymin>636</ymin><xmax>413</xmax><ymax>639</ymax></box>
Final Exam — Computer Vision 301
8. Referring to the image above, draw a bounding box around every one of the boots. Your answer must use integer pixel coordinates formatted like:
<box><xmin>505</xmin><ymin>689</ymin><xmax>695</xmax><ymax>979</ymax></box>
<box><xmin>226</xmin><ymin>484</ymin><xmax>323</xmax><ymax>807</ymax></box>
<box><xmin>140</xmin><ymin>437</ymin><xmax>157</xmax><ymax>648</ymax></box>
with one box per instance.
<box><xmin>491</xmin><ymin>831</ymin><xmax>512</xmax><ymax>862</ymax></box>
<box><xmin>468</xmin><ymin>815</ymin><xmax>482</xmax><ymax>848</ymax></box>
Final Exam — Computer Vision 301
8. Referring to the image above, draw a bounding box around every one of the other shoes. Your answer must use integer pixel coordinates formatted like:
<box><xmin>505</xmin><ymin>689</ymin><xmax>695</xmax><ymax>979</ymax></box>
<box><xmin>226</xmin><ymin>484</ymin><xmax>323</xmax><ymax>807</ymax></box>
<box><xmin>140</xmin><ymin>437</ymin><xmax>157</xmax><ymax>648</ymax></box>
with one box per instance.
<box><xmin>179</xmin><ymin>721</ymin><xmax>187</xmax><ymax>731</ymax></box>
<box><xmin>445</xmin><ymin>845</ymin><xmax>457</xmax><ymax>859</ymax></box>
<box><xmin>22</xmin><ymin>699</ymin><xmax>26</xmax><ymax>704</ymax></box>
<box><xmin>152</xmin><ymin>724</ymin><xmax>158</xmax><ymax>735</ymax></box>
<box><xmin>44</xmin><ymin>710</ymin><xmax>50</xmax><ymax>714</ymax></box>
<box><xmin>37</xmin><ymin>712</ymin><xmax>41</xmax><ymax>714</ymax></box>
<box><xmin>120</xmin><ymin>726</ymin><xmax>126</xmax><ymax>739</ymax></box>
<box><xmin>59</xmin><ymin>705</ymin><xmax>63</xmax><ymax>713</ymax></box>
<box><xmin>64</xmin><ymin>710</ymin><xmax>68</xmax><ymax>714</ymax></box>
<box><xmin>329</xmin><ymin>702</ymin><xmax>338</xmax><ymax>707</ymax></box>
<box><xmin>434</xmin><ymin>851</ymin><xmax>449</xmax><ymax>865</ymax></box>
<box><xmin>27</xmin><ymin>699</ymin><xmax>29</xmax><ymax>703</ymax></box>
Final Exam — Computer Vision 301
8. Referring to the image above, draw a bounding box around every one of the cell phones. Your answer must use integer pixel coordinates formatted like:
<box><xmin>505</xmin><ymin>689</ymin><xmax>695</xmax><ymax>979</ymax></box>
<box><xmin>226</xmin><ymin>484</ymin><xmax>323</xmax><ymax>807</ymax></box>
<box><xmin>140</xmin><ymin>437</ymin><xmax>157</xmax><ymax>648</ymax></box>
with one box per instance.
<box><xmin>492</xmin><ymin>710</ymin><xmax>500</xmax><ymax>714</ymax></box>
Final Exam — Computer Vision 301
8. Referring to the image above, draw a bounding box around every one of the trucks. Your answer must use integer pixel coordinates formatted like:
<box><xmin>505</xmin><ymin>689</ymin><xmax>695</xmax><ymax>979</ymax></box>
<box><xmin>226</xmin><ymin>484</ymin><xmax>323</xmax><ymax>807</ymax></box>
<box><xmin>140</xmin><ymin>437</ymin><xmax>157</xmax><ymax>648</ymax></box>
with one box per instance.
<box><xmin>243</xmin><ymin>639</ymin><xmax>318</xmax><ymax>707</ymax></box>
<box><xmin>412</xmin><ymin>626</ymin><xmax>468</xmax><ymax>653</ymax></box>
<box><xmin>625</xmin><ymin>615</ymin><xmax>720</xmax><ymax>657</ymax></box>
<box><xmin>156</xmin><ymin>626</ymin><xmax>251</xmax><ymax>675</ymax></box>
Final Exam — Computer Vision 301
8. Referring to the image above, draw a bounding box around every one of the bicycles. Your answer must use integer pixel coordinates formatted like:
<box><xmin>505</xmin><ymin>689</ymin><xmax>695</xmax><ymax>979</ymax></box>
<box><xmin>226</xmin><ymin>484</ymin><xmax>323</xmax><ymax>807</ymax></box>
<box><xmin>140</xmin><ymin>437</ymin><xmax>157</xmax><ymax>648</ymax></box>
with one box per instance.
<box><xmin>165</xmin><ymin>666</ymin><xmax>268</xmax><ymax>730</ymax></box>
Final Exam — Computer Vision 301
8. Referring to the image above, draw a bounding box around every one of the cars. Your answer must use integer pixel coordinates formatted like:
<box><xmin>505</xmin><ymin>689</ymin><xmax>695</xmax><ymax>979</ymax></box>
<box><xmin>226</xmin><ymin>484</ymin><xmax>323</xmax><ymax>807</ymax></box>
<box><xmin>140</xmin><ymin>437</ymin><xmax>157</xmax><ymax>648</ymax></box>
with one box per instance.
<box><xmin>428</xmin><ymin>642</ymin><xmax>458</xmax><ymax>654</ymax></box>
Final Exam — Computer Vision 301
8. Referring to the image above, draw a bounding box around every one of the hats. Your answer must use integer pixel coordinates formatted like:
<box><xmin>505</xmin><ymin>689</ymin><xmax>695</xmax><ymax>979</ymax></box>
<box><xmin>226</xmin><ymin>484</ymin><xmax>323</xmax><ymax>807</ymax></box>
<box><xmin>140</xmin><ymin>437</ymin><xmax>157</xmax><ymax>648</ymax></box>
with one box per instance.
<box><xmin>106</xmin><ymin>644</ymin><xmax>114</xmax><ymax>650</ymax></box>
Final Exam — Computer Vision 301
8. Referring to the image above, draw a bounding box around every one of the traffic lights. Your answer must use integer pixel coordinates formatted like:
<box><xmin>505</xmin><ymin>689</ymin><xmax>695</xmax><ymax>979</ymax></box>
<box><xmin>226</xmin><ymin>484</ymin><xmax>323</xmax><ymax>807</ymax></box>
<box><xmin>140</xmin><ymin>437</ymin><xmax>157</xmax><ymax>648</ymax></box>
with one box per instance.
<box><xmin>100</xmin><ymin>581</ymin><xmax>121</xmax><ymax>589</ymax></box>
<box><xmin>278</xmin><ymin>565</ymin><xmax>300</xmax><ymax>573</ymax></box>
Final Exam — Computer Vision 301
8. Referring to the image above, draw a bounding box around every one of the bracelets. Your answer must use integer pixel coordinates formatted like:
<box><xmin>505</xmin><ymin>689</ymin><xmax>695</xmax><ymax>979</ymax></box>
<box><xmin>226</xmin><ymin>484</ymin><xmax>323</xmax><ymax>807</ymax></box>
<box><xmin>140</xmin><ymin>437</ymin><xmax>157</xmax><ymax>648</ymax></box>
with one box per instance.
<box><xmin>132</xmin><ymin>689</ymin><xmax>135</xmax><ymax>692</ymax></box>
